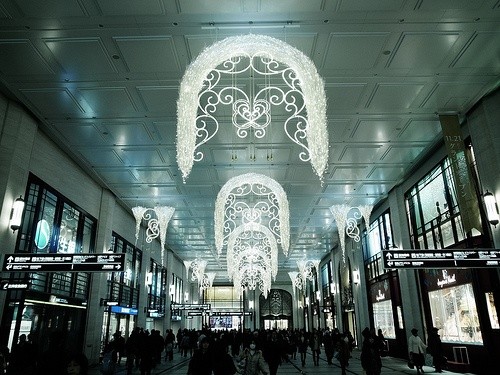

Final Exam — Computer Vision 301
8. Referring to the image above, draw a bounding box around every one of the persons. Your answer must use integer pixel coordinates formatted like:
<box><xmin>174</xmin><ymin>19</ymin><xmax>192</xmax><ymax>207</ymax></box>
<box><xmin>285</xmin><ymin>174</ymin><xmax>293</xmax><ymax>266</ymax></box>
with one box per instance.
<box><xmin>409</xmin><ymin>328</ymin><xmax>428</xmax><ymax>375</ymax></box>
<box><xmin>426</xmin><ymin>328</ymin><xmax>448</xmax><ymax>373</ymax></box>
<box><xmin>0</xmin><ymin>327</ymin><xmax>387</xmax><ymax>375</ymax></box>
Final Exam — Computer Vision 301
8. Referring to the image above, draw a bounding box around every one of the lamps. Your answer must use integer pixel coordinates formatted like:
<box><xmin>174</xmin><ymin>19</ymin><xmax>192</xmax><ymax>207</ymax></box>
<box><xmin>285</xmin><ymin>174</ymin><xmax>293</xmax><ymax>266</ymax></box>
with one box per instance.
<box><xmin>483</xmin><ymin>189</ymin><xmax>499</xmax><ymax>228</ymax></box>
<box><xmin>296</xmin><ymin>260</ymin><xmax>321</xmax><ymax>297</ymax></box>
<box><xmin>183</xmin><ymin>172</ymin><xmax>290</xmax><ymax>301</ymax></box>
<box><xmin>147</xmin><ymin>270</ymin><xmax>153</xmax><ymax>289</ymax></box>
<box><xmin>131</xmin><ymin>202</ymin><xmax>175</xmax><ymax>267</ymax></box>
<box><xmin>329</xmin><ymin>205</ymin><xmax>374</xmax><ymax>264</ymax></box>
<box><xmin>10</xmin><ymin>195</ymin><xmax>25</xmax><ymax>233</ymax></box>
<box><xmin>175</xmin><ymin>21</ymin><xmax>330</xmax><ymax>187</ymax></box>
<box><xmin>288</xmin><ymin>271</ymin><xmax>315</xmax><ymax>299</ymax></box>
<box><xmin>352</xmin><ymin>268</ymin><xmax>358</xmax><ymax>286</ymax></box>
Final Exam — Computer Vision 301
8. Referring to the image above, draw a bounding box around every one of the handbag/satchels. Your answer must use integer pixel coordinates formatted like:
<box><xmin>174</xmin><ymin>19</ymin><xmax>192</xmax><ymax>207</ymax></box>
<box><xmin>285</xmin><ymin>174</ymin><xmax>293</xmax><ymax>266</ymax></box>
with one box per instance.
<box><xmin>442</xmin><ymin>356</ymin><xmax>449</xmax><ymax>370</ymax></box>
<box><xmin>408</xmin><ymin>355</ymin><xmax>414</xmax><ymax>369</ymax></box>
<box><xmin>424</xmin><ymin>354</ymin><xmax>433</xmax><ymax>367</ymax></box>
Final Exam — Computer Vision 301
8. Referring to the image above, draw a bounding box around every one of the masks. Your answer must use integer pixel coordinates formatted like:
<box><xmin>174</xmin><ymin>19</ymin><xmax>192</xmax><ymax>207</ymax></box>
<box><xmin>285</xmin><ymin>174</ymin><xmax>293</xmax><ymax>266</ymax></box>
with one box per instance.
<box><xmin>250</xmin><ymin>343</ymin><xmax>256</xmax><ymax>349</ymax></box>
